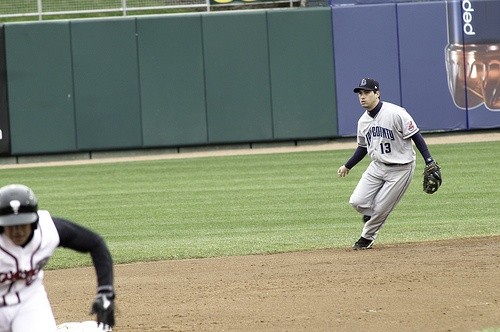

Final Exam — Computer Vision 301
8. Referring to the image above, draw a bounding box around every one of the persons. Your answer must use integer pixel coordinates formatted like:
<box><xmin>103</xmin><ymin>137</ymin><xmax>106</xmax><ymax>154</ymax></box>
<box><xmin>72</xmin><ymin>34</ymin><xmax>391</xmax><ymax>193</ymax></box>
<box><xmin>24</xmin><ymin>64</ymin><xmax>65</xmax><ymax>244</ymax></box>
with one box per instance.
<box><xmin>0</xmin><ymin>183</ymin><xmax>115</xmax><ymax>332</ymax></box>
<box><xmin>337</xmin><ymin>78</ymin><xmax>442</xmax><ymax>250</ymax></box>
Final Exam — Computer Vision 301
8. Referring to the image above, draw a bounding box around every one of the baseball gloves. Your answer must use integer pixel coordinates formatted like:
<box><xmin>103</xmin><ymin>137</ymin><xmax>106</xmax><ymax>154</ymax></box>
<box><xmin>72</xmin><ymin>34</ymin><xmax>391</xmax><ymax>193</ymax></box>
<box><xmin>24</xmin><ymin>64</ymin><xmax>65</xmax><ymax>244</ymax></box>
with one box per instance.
<box><xmin>422</xmin><ymin>157</ymin><xmax>443</xmax><ymax>194</ymax></box>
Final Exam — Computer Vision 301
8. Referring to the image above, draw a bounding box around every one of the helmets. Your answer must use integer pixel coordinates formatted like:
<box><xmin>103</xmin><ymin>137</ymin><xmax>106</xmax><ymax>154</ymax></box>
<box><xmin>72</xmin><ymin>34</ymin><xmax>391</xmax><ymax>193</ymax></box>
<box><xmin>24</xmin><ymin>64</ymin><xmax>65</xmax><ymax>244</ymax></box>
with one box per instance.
<box><xmin>0</xmin><ymin>184</ymin><xmax>39</xmax><ymax>234</ymax></box>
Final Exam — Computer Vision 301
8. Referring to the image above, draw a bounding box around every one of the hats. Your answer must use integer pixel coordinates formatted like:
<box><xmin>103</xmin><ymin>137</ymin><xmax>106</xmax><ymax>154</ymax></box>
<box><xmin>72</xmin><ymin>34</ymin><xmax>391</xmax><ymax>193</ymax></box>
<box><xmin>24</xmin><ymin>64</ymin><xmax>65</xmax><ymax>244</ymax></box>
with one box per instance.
<box><xmin>353</xmin><ymin>78</ymin><xmax>379</xmax><ymax>93</ymax></box>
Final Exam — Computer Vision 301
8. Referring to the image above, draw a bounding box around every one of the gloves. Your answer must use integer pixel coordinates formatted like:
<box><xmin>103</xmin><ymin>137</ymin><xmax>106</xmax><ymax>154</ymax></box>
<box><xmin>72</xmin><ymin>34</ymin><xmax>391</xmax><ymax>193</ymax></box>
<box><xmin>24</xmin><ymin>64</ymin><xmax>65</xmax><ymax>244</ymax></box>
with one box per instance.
<box><xmin>88</xmin><ymin>290</ymin><xmax>115</xmax><ymax>330</ymax></box>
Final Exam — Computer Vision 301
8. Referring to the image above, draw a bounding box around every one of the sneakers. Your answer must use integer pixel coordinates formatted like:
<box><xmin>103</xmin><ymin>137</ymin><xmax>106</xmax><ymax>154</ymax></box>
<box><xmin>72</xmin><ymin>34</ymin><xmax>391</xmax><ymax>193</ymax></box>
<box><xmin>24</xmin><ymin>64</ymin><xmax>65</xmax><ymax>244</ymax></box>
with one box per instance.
<box><xmin>364</xmin><ymin>215</ymin><xmax>371</xmax><ymax>222</ymax></box>
<box><xmin>352</xmin><ymin>237</ymin><xmax>375</xmax><ymax>249</ymax></box>
